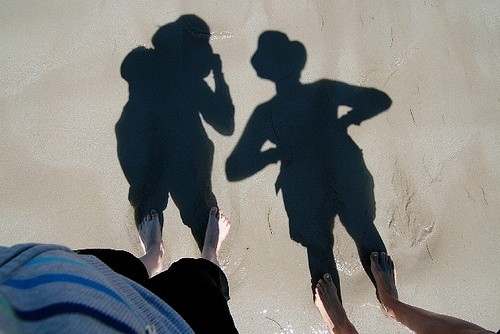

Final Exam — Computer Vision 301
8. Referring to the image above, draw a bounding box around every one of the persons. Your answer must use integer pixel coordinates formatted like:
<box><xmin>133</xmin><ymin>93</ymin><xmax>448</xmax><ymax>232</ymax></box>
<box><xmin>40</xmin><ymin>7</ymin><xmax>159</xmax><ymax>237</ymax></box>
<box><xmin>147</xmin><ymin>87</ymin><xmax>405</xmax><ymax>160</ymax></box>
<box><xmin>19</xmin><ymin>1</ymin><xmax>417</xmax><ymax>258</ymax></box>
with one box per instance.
<box><xmin>0</xmin><ymin>207</ymin><xmax>239</xmax><ymax>334</ymax></box>
<box><xmin>315</xmin><ymin>249</ymin><xmax>500</xmax><ymax>334</ymax></box>
